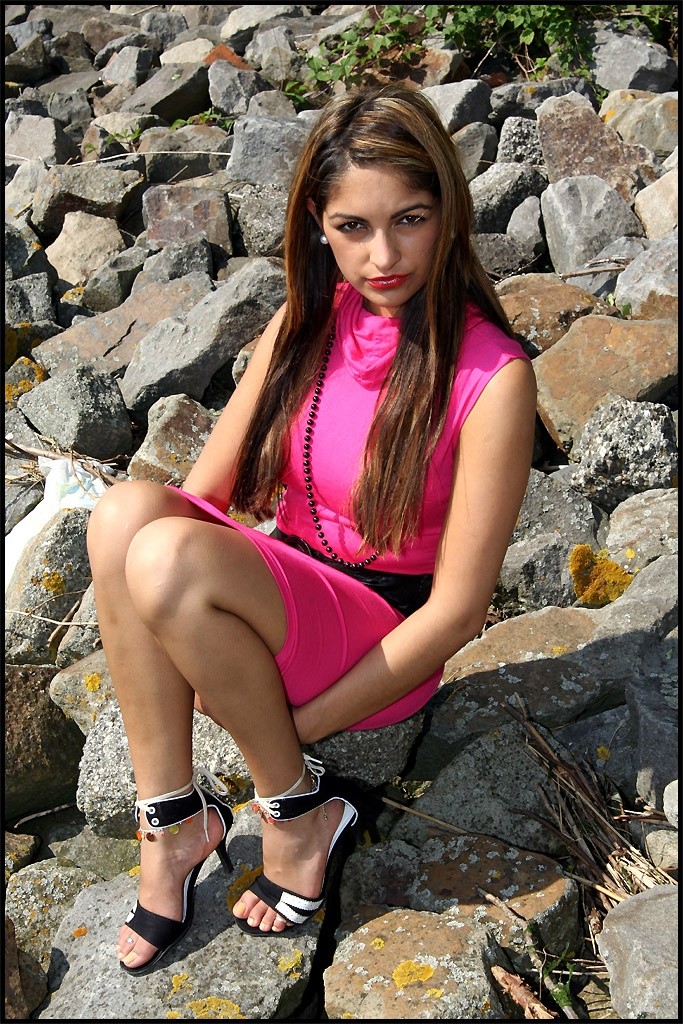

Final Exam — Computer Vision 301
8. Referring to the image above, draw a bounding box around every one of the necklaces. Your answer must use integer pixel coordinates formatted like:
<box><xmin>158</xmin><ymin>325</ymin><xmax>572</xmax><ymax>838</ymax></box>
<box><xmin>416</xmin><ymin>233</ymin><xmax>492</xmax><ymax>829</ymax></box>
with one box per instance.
<box><xmin>304</xmin><ymin>319</ymin><xmax>390</xmax><ymax>570</ymax></box>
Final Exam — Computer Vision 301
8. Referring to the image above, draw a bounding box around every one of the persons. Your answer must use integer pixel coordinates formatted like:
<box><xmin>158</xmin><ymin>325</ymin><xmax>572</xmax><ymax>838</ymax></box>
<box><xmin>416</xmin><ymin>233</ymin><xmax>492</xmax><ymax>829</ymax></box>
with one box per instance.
<box><xmin>87</xmin><ymin>85</ymin><xmax>538</xmax><ymax>975</ymax></box>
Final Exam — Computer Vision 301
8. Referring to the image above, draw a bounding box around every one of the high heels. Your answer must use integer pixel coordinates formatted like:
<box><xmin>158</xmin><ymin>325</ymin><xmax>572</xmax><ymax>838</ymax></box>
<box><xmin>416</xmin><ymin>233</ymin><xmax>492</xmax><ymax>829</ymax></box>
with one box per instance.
<box><xmin>235</xmin><ymin>753</ymin><xmax>384</xmax><ymax>937</ymax></box>
<box><xmin>118</xmin><ymin>770</ymin><xmax>233</xmax><ymax>975</ymax></box>
